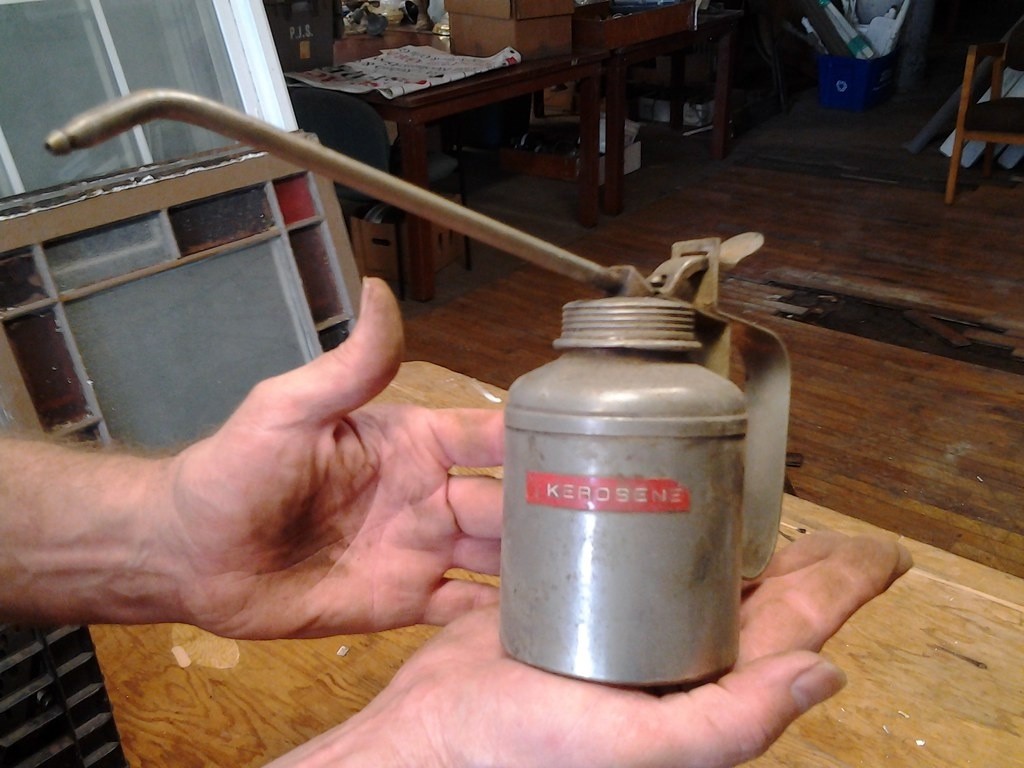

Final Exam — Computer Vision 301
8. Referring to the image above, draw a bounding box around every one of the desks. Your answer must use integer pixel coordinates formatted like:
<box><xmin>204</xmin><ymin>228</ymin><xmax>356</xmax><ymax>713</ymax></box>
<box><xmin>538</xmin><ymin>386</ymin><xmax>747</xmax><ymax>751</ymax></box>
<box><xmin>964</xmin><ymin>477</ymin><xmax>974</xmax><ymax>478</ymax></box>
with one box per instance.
<box><xmin>88</xmin><ymin>361</ymin><xmax>1024</xmax><ymax>768</ymax></box>
<box><xmin>287</xmin><ymin>30</ymin><xmax>611</xmax><ymax>302</ymax></box>
<box><xmin>385</xmin><ymin>10</ymin><xmax>744</xmax><ymax>216</ymax></box>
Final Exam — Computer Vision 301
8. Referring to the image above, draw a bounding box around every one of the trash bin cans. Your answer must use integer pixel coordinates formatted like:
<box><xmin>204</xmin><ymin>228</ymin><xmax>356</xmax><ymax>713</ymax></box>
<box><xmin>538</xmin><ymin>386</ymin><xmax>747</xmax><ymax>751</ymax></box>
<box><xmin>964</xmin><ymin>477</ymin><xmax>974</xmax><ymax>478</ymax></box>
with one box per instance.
<box><xmin>817</xmin><ymin>45</ymin><xmax>901</xmax><ymax>111</ymax></box>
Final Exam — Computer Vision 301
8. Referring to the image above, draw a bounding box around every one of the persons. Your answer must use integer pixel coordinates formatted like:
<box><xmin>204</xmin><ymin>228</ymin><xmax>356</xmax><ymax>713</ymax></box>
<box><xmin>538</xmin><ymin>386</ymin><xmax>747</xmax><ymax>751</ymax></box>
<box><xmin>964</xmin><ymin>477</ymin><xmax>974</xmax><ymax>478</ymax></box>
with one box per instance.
<box><xmin>1</xmin><ymin>274</ymin><xmax>914</xmax><ymax>768</ymax></box>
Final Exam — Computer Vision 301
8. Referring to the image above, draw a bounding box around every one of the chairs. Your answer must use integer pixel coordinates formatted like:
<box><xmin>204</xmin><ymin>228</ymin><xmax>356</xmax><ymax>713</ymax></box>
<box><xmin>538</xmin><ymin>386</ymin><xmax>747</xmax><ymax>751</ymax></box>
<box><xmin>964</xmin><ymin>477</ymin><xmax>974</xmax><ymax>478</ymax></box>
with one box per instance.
<box><xmin>944</xmin><ymin>18</ymin><xmax>1024</xmax><ymax>206</ymax></box>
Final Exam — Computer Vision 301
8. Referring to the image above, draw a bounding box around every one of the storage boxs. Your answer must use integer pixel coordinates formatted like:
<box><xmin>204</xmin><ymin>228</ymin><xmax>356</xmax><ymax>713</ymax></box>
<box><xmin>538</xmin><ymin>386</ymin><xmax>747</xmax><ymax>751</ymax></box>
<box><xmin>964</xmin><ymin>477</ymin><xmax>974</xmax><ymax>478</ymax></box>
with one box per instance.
<box><xmin>818</xmin><ymin>50</ymin><xmax>896</xmax><ymax>112</ymax></box>
<box><xmin>445</xmin><ymin>0</ymin><xmax>575</xmax><ymax>60</ymax></box>
<box><xmin>572</xmin><ymin>0</ymin><xmax>694</xmax><ymax>49</ymax></box>
<box><xmin>349</xmin><ymin>194</ymin><xmax>465</xmax><ymax>279</ymax></box>
